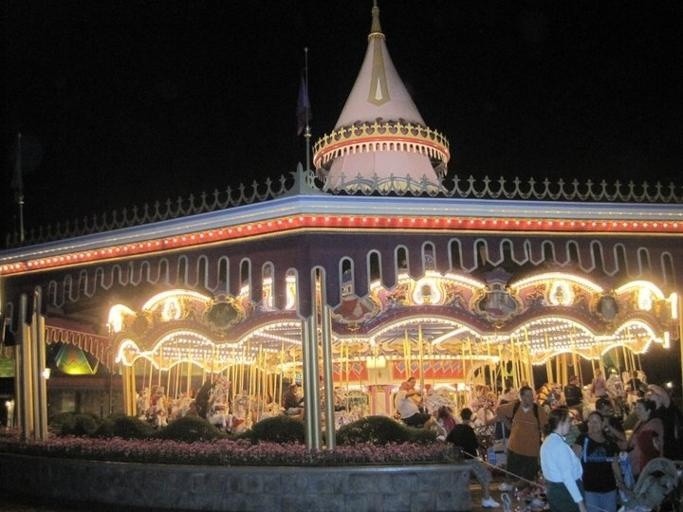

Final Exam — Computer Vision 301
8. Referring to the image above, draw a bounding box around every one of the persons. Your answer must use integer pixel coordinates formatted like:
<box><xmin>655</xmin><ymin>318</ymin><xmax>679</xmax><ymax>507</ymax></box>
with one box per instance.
<box><xmin>395</xmin><ymin>377</ymin><xmax>430</xmax><ymax>426</ymax></box>
<box><xmin>285</xmin><ymin>385</ymin><xmax>305</xmax><ymax>417</ymax></box>
<box><xmin>496</xmin><ymin>370</ymin><xmax>683</xmax><ymax>512</ymax></box>
<box><xmin>197</xmin><ymin>381</ymin><xmax>216</xmax><ymax>419</ymax></box>
<box><xmin>151</xmin><ymin>385</ymin><xmax>160</xmax><ymax>413</ymax></box>
<box><xmin>439</xmin><ymin>406</ymin><xmax>500</xmax><ymax>507</ymax></box>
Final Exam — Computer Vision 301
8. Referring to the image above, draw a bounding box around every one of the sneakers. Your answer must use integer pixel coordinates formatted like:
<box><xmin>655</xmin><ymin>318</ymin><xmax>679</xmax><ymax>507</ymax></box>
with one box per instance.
<box><xmin>479</xmin><ymin>495</ymin><xmax>503</xmax><ymax>508</ymax></box>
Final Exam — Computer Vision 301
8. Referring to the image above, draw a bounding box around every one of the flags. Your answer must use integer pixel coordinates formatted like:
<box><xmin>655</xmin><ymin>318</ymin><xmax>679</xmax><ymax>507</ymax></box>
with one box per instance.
<box><xmin>294</xmin><ymin>52</ymin><xmax>313</xmax><ymax>136</ymax></box>
<box><xmin>8</xmin><ymin>137</ymin><xmax>25</xmax><ymax>202</ymax></box>
